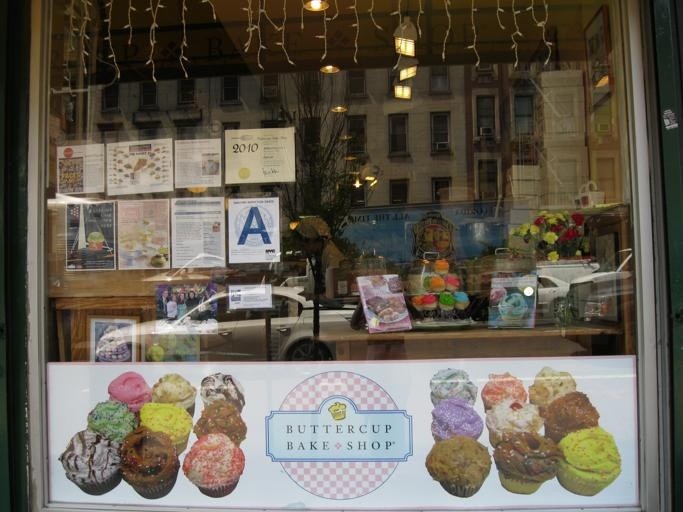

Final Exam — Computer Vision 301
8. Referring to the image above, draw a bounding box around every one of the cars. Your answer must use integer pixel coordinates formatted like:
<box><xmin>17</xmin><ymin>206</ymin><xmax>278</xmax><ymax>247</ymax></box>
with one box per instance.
<box><xmin>89</xmin><ymin>283</ymin><xmax>360</xmax><ymax>362</ymax></box>
<box><xmin>136</xmin><ymin>251</ymin><xmax>245</xmax><ymax>283</ymax></box>
<box><xmin>506</xmin><ymin>251</ymin><xmax>633</xmax><ymax>353</ymax></box>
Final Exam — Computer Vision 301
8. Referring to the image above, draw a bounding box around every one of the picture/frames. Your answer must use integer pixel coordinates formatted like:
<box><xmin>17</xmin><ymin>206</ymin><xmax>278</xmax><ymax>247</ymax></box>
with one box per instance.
<box><xmin>83</xmin><ymin>313</ymin><xmax>142</xmax><ymax>364</ymax></box>
<box><xmin>579</xmin><ymin>0</ymin><xmax>616</xmax><ymax>117</ymax></box>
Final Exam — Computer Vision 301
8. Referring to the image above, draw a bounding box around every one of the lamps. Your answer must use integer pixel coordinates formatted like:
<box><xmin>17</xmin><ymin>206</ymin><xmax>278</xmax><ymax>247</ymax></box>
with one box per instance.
<box><xmin>392</xmin><ymin>1</ymin><xmax>418</xmax><ymax>102</ymax></box>
<box><xmin>301</xmin><ymin>0</ymin><xmax>330</xmax><ymax>12</ymax></box>
<box><xmin>329</xmin><ymin>98</ymin><xmax>348</xmax><ymax>113</ymax></box>
<box><xmin>316</xmin><ymin>59</ymin><xmax>345</xmax><ymax>74</ymax></box>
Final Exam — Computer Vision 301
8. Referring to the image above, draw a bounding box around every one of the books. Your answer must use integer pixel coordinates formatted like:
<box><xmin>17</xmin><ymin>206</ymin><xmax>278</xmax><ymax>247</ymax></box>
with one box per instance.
<box><xmin>487</xmin><ymin>275</ymin><xmax>536</xmax><ymax>329</ymax></box>
<box><xmin>355</xmin><ymin>273</ymin><xmax>412</xmax><ymax>334</ymax></box>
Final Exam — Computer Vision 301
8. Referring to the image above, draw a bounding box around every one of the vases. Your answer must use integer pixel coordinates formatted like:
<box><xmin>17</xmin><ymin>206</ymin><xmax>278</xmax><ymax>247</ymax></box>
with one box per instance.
<box><xmin>532</xmin><ymin>254</ymin><xmax>600</xmax><ymax>322</ymax></box>
<box><xmin>551</xmin><ymin>286</ymin><xmax>574</xmax><ymax>327</ymax></box>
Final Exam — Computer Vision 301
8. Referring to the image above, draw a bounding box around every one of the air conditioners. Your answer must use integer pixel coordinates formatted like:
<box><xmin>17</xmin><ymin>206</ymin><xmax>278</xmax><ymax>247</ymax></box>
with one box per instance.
<box><xmin>434</xmin><ymin>141</ymin><xmax>450</xmax><ymax>152</ymax></box>
<box><xmin>478</xmin><ymin>126</ymin><xmax>494</xmax><ymax>137</ymax></box>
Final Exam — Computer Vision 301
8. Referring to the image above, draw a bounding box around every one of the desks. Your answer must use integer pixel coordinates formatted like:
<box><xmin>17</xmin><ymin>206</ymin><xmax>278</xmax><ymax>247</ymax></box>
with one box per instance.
<box><xmin>311</xmin><ymin>317</ymin><xmax>625</xmax><ymax>361</ymax></box>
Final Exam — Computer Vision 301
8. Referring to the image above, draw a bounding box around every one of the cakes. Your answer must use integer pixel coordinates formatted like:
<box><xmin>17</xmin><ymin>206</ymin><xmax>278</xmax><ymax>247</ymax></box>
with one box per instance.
<box><xmin>145</xmin><ymin>327</ymin><xmax>198</xmax><ymax>362</ymax></box>
<box><xmin>60</xmin><ymin>371</ymin><xmax>247</xmax><ymax>499</ymax></box>
<box><xmin>425</xmin><ymin>367</ymin><xmax>621</xmax><ymax>498</ymax></box>
<box><xmin>88</xmin><ymin>232</ymin><xmax>104</xmax><ymax>251</ymax></box>
<box><xmin>490</xmin><ymin>274</ymin><xmax>536</xmax><ymax>322</ymax></box>
<box><xmin>413</xmin><ymin>260</ymin><xmax>470</xmax><ymax>311</ymax></box>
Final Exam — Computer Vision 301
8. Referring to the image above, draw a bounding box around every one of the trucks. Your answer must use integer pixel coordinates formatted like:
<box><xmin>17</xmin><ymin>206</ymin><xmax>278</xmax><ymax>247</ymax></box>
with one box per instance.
<box><xmin>255</xmin><ymin>195</ymin><xmax>536</xmax><ymax>323</ymax></box>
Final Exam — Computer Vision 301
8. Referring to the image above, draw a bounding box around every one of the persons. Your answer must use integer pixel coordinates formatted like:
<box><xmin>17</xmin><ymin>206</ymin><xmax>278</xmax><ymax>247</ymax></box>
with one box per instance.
<box><xmin>280</xmin><ymin>221</ymin><xmax>342</xmax><ymax>297</ymax></box>
<box><xmin>350</xmin><ymin>256</ymin><xmax>421</xmax><ymax>360</ymax></box>
<box><xmin>157</xmin><ymin>288</ymin><xmax>214</xmax><ymax>321</ymax></box>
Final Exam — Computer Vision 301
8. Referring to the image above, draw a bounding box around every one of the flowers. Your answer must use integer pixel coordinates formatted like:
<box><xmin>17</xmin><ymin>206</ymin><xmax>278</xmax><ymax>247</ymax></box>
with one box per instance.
<box><xmin>506</xmin><ymin>203</ymin><xmax>597</xmax><ymax>268</ymax></box>
